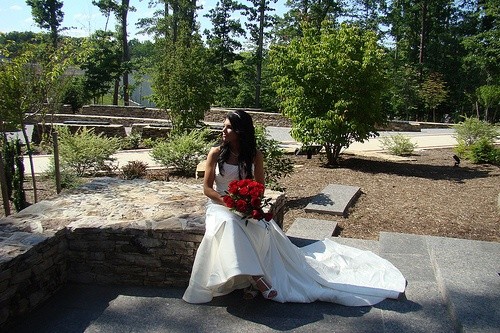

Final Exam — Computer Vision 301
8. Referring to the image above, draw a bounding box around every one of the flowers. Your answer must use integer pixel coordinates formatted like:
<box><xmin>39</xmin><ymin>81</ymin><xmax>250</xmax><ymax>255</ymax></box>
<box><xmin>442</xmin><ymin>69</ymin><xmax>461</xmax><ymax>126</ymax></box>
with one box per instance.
<box><xmin>220</xmin><ymin>179</ymin><xmax>275</xmax><ymax>227</ymax></box>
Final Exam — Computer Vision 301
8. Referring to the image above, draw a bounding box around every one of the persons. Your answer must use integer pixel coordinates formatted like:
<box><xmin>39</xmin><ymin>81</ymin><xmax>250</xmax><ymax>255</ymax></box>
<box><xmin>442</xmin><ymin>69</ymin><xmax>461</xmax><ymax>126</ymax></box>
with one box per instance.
<box><xmin>203</xmin><ymin>109</ymin><xmax>278</xmax><ymax>300</ymax></box>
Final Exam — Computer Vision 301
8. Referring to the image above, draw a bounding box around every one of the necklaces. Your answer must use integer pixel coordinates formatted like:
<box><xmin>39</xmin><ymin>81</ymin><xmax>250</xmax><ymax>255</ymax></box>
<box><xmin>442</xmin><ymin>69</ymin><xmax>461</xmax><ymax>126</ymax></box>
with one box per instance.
<box><xmin>230</xmin><ymin>149</ymin><xmax>238</xmax><ymax>157</ymax></box>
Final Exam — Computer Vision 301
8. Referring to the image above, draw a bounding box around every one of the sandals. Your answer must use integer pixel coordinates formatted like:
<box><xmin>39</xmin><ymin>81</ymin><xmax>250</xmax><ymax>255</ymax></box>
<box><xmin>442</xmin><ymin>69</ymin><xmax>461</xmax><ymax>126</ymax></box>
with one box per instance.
<box><xmin>256</xmin><ymin>277</ymin><xmax>277</xmax><ymax>299</ymax></box>
<box><xmin>244</xmin><ymin>291</ymin><xmax>259</xmax><ymax>299</ymax></box>
